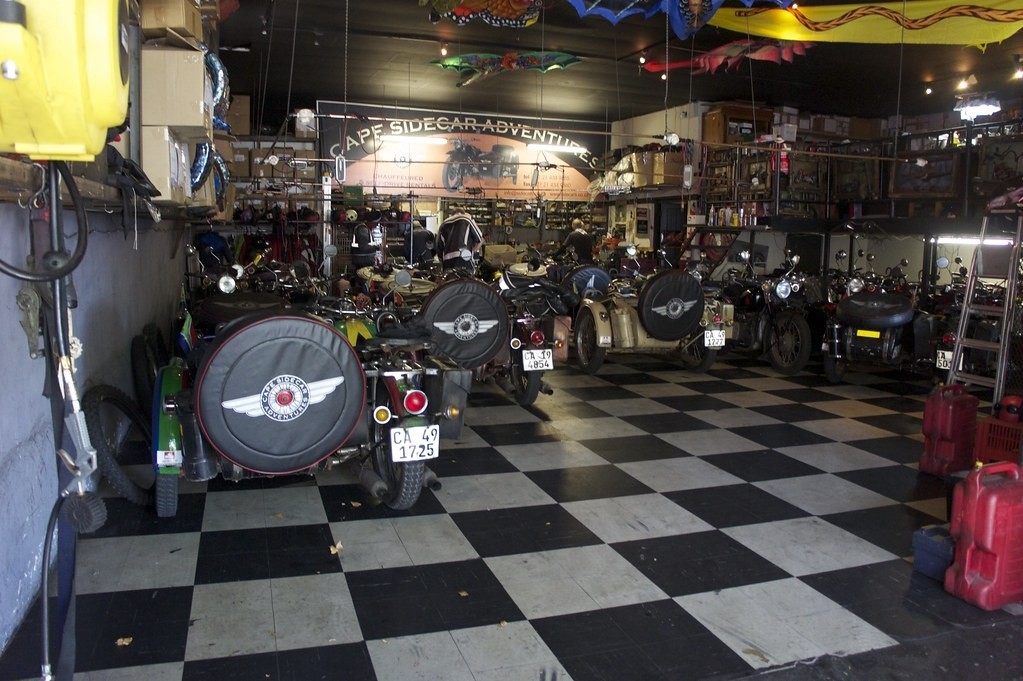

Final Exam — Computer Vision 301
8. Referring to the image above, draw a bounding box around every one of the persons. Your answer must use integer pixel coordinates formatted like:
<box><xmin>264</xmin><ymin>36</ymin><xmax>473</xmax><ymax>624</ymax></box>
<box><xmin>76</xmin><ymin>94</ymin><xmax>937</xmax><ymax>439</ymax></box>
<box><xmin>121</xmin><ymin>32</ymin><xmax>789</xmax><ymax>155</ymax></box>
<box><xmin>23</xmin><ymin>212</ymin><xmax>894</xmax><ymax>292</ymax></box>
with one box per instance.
<box><xmin>435</xmin><ymin>207</ymin><xmax>485</xmax><ymax>279</ymax></box>
<box><xmin>404</xmin><ymin>220</ymin><xmax>436</xmax><ymax>282</ymax></box>
<box><xmin>562</xmin><ymin>218</ymin><xmax>592</xmax><ymax>263</ymax></box>
<box><xmin>351</xmin><ymin>211</ymin><xmax>381</xmax><ymax>304</ymax></box>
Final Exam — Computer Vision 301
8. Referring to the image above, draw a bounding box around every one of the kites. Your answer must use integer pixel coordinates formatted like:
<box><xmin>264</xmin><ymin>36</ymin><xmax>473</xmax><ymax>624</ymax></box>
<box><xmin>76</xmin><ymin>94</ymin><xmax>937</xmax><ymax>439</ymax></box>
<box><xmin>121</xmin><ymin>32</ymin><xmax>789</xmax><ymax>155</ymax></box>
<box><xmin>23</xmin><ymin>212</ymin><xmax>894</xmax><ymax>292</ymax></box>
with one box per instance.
<box><xmin>567</xmin><ymin>0</ymin><xmax>796</xmax><ymax>41</ymax></box>
<box><xmin>418</xmin><ymin>0</ymin><xmax>542</xmax><ymax>28</ymax></box>
<box><xmin>708</xmin><ymin>0</ymin><xmax>1023</xmax><ymax>45</ymax></box>
<box><xmin>430</xmin><ymin>51</ymin><xmax>583</xmax><ymax>87</ymax></box>
<box><xmin>641</xmin><ymin>37</ymin><xmax>817</xmax><ymax>76</ymax></box>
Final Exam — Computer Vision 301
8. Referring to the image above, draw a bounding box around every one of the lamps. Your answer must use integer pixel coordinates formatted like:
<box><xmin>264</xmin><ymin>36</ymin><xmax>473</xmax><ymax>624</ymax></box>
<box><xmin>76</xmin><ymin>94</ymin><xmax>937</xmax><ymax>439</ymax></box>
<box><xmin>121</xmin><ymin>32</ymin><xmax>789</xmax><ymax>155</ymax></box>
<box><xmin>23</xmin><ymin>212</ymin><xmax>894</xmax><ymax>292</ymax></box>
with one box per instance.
<box><xmin>922</xmin><ymin>236</ymin><xmax>1023</xmax><ymax>245</ymax></box>
<box><xmin>526</xmin><ymin>144</ymin><xmax>587</xmax><ymax>154</ymax></box>
<box><xmin>953</xmin><ymin>90</ymin><xmax>1001</xmax><ymax>122</ymax></box>
<box><xmin>378</xmin><ymin>134</ymin><xmax>447</xmax><ymax>144</ymax></box>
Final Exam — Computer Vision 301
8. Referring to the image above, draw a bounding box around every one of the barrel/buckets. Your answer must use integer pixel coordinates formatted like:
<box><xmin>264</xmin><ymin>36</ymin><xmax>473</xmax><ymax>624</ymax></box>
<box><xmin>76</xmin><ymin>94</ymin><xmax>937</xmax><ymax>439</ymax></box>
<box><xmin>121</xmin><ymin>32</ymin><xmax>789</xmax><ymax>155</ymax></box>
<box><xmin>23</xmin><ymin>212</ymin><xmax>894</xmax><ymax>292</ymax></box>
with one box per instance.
<box><xmin>944</xmin><ymin>461</ymin><xmax>1023</xmax><ymax>611</ymax></box>
<box><xmin>919</xmin><ymin>383</ymin><xmax>979</xmax><ymax>477</ymax></box>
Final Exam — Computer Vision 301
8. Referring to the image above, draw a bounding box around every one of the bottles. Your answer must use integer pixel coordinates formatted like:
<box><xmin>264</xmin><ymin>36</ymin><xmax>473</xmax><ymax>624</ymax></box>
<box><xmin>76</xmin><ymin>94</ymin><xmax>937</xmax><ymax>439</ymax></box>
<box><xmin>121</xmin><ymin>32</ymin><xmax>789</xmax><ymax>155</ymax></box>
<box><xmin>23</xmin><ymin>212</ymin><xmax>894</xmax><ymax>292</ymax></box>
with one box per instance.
<box><xmin>709</xmin><ymin>204</ymin><xmax>745</xmax><ymax>226</ymax></box>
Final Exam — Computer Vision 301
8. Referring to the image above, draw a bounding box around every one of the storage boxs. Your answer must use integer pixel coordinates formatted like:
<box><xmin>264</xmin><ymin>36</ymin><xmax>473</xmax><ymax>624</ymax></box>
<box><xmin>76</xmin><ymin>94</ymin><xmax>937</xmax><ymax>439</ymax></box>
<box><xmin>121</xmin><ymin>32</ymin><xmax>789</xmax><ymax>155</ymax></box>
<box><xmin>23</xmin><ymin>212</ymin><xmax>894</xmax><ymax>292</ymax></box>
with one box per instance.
<box><xmin>973</xmin><ymin>414</ymin><xmax>1023</xmax><ymax>471</ymax></box>
<box><xmin>911</xmin><ymin>523</ymin><xmax>956</xmax><ymax>582</ymax></box>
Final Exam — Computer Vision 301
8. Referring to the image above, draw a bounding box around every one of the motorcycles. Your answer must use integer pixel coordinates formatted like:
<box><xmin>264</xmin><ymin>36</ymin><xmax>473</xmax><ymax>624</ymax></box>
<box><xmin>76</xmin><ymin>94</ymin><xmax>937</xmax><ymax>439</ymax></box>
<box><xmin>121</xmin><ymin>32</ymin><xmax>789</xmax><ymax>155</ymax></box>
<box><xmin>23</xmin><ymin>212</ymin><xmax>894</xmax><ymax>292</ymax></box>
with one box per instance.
<box><xmin>149</xmin><ymin>241</ymin><xmax>1023</xmax><ymax>519</ymax></box>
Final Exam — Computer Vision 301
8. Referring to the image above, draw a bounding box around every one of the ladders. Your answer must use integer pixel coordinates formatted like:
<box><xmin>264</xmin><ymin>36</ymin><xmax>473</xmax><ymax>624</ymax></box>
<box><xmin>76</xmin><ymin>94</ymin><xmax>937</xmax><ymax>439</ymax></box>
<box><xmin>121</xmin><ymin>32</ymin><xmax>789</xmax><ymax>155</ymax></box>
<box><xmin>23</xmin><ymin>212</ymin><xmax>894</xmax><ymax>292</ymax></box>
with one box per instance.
<box><xmin>943</xmin><ymin>217</ymin><xmax>1022</xmax><ymax>403</ymax></box>
